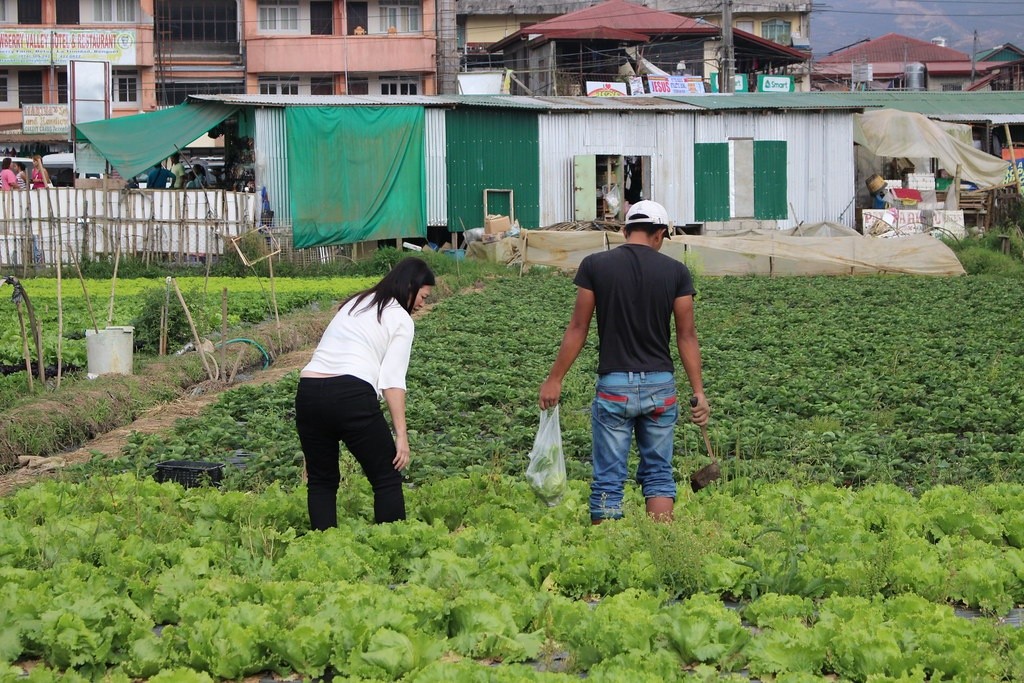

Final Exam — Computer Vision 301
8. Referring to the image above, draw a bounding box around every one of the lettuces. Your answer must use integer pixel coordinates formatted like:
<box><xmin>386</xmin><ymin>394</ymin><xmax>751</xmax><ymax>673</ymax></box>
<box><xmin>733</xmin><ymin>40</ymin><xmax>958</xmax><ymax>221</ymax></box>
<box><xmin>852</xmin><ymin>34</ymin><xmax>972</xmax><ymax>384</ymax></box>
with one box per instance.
<box><xmin>529</xmin><ymin>447</ymin><xmax>564</xmax><ymax>502</ymax></box>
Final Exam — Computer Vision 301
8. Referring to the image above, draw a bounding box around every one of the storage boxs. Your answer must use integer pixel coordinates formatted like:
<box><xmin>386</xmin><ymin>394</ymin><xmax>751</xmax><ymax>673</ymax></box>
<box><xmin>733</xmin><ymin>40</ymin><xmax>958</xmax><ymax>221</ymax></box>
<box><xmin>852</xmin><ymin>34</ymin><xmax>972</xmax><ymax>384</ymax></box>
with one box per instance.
<box><xmin>884</xmin><ymin>158</ymin><xmax>935</xmax><ymax>190</ymax></box>
<box><xmin>155</xmin><ymin>459</ymin><xmax>226</xmax><ymax>490</ymax></box>
<box><xmin>480</xmin><ymin>232</ymin><xmax>501</xmax><ymax>243</ymax></box>
<box><xmin>484</xmin><ymin>214</ymin><xmax>512</xmax><ymax>236</ymax></box>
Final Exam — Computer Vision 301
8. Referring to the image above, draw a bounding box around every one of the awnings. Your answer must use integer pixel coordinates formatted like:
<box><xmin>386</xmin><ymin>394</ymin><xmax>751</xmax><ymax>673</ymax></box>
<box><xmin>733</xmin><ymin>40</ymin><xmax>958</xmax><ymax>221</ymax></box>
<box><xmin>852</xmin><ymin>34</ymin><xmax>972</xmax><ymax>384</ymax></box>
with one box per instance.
<box><xmin>71</xmin><ymin>98</ymin><xmax>245</xmax><ymax>183</ymax></box>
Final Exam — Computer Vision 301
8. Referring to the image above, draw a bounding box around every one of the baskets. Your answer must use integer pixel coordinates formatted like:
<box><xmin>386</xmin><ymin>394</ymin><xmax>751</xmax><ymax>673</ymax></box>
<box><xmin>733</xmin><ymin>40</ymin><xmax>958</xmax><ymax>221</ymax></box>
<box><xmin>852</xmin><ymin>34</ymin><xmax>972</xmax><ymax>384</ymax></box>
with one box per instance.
<box><xmin>155</xmin><ymin>460</ymin><xmax>225</xmax><ymax>488</ymax></box>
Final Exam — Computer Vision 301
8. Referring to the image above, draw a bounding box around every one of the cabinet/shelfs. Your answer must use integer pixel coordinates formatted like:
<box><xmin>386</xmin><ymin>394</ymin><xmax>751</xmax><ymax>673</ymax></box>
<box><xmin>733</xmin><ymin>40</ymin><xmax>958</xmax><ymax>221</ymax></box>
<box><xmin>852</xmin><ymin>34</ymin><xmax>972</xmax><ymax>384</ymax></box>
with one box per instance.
<box><xmin>596</xmin><ymin>155</ymin><xmax>624</xmax><ymax>222</ymax></box>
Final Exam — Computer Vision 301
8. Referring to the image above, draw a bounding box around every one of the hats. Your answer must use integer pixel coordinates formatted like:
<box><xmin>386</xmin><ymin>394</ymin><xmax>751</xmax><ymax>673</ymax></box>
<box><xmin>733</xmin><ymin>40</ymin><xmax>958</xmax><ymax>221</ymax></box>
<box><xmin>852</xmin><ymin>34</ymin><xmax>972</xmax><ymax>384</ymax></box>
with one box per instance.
<box><xmin>625</xmin><ymin>200</ymin><xmax>671</xmax><ymax>240</ymax></box>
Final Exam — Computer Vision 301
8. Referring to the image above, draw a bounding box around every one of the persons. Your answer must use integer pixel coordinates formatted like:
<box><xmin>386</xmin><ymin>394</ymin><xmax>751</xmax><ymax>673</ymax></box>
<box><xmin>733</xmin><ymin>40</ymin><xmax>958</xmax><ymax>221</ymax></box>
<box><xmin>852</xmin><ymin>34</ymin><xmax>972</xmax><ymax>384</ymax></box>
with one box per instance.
<box><xmin>292</xmin><ymin>256</ymin><xmax>437</xmax><ymax>538</ymax></box>
<box><xmin>0</xmin><ymin>155</ymin><xmax>50</xmax><ymax>191</ymax></box>
<box><xmin>537</xmin><ymin>198</ymin><xmax>712</xmax><ymax>530</ymax></box>
<box><xmin>126</xmin><ymin>152</ymin><xmax>207</xmax><ymax>189</ymax></box>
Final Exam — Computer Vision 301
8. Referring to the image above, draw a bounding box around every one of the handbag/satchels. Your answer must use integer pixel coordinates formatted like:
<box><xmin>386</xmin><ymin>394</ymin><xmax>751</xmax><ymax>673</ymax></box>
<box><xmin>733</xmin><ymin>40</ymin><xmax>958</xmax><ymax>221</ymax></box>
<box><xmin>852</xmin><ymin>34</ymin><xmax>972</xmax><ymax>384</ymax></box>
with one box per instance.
<box><xmin>46</xmin><ymin>179</ymin><xmax>53</xmax><ymax>188</ymax></box>
<box><xmin>525</xmin><ymin>405</ymin><xmax>567</xmax><ymax>508</ymax></box>
